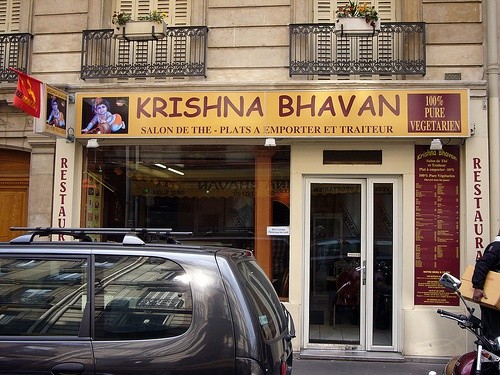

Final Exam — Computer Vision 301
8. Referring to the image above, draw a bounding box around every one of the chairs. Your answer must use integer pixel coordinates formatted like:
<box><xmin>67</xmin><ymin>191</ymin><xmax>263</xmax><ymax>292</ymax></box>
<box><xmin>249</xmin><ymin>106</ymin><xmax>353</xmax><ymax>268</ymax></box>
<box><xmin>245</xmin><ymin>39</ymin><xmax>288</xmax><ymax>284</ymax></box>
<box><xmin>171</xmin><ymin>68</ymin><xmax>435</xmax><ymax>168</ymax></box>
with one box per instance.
<box><xmin>333</xmin><ymin>269</ymin><xmax>360</xmax><ymax>330</ymax></box>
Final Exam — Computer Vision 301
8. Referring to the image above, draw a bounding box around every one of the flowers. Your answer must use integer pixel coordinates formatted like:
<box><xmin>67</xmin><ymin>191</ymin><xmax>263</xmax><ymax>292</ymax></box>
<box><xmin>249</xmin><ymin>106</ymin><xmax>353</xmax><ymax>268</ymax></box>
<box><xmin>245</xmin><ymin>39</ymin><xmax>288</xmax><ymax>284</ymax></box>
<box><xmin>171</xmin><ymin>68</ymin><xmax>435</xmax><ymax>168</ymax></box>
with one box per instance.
<box><xmin>111</xmin><ymin>10</ymin><xmax>167</xmax><ymax>25</ymax></box>
<box><xmin>336</xmin><ymin>0</ymin><xmax>378</xmax><ymax>24</ymax></box>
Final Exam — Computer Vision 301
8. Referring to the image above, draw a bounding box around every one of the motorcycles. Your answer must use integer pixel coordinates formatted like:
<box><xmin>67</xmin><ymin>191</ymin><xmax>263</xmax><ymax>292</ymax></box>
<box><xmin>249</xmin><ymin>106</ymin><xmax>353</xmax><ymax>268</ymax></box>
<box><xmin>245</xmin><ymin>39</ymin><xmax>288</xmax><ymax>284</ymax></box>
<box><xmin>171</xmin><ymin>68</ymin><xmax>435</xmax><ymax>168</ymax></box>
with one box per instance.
<box><xmin>436</xmin><ymin>272</ymin><xmax>500</xmax><ymax>375</ymax></box>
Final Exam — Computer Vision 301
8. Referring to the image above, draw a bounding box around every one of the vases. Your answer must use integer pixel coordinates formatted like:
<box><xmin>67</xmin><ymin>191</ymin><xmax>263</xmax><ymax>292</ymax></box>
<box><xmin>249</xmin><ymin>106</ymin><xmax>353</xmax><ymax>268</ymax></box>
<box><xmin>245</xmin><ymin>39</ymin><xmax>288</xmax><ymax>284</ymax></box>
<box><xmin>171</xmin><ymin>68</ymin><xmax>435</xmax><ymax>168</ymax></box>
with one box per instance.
<box><xmin>114</xmin><ymin>21</ymin><xmax>167</xmax><ymax>40</ymax></box>
<box><xmin>334</xmin><ymin>18</ymin><xmax>381</xmax><ymax>37</ymax></box>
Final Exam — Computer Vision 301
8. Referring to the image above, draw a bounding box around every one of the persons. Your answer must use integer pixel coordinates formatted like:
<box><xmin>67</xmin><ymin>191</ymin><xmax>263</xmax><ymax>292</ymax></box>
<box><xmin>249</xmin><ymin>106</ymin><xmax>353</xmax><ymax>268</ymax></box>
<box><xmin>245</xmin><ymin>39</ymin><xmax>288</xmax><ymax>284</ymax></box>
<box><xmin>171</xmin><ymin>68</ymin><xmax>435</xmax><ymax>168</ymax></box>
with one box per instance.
<box><xmin>471</xmin><ymin>230</ymin><xmax>500</xmax><ymax>338</ymax></box>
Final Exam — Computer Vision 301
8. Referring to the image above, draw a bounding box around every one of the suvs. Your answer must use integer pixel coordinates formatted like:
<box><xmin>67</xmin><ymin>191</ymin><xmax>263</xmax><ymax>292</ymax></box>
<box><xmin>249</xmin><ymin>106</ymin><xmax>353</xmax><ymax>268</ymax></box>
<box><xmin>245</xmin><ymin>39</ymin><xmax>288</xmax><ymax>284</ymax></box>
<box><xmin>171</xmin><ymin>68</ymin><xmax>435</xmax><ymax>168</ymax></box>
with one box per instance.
<box><xmin>0</xmin><ymin>226</ymin><xmax>297</xmax><ymax>375</ymax></box>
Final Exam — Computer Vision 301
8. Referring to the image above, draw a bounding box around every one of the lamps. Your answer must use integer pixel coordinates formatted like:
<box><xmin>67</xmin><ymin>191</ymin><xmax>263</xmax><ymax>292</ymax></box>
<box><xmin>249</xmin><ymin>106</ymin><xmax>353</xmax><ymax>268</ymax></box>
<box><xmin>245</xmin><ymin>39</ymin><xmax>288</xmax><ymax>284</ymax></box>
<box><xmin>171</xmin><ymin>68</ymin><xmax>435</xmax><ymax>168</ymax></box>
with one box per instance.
<box><xmin>264</xmin><ymin>138</ymin><xmax>276</xmax><ymax>147</ymax></box>
<box><xmin>86</xmin><ymin>139</ymin><xmax>99</xmax><ymax>148</ymax></box>
<box><xmin>430</xmin><ymin>139</ymin><xmax>442</xmax><ymax>150</ymax></box>
<box><xmin>66</xmin><ymin>127</ymin><xmax>75</xmax><ymax>143</ymax></box>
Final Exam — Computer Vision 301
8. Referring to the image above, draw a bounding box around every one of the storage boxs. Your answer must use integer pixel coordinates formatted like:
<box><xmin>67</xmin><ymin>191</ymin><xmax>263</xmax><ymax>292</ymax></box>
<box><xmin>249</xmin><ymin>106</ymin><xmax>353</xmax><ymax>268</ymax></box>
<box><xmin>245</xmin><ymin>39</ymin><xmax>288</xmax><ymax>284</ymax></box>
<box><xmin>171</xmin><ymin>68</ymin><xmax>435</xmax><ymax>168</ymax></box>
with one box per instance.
<box><xmin>456</xmin><ymin>266</ymin><xmax>500</xmax><ymax>311</ymax></box>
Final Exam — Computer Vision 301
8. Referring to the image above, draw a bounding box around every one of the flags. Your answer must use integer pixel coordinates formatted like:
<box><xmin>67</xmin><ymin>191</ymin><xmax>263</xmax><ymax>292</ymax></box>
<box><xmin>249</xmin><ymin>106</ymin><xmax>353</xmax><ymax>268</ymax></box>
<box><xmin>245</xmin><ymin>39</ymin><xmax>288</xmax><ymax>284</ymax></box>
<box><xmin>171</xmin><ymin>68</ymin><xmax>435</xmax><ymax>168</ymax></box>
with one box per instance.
<box><xmin>12</xmin><ymin>71</ymin><xmax>43</xmax><ymax>118</ymax></box>
